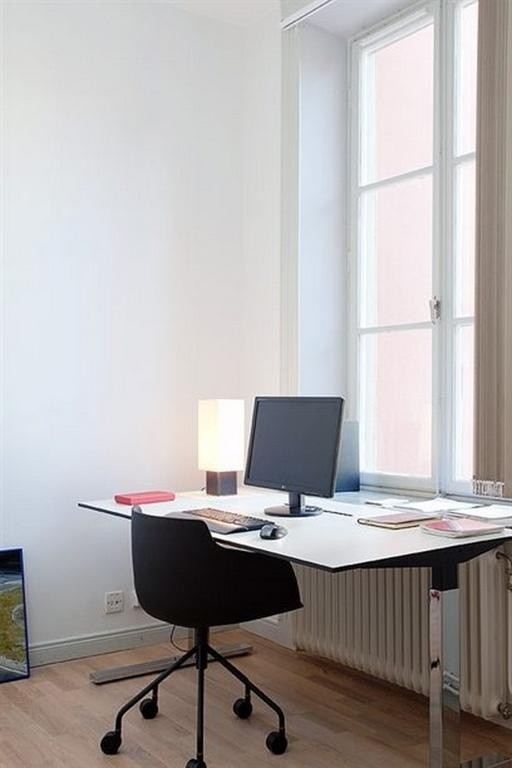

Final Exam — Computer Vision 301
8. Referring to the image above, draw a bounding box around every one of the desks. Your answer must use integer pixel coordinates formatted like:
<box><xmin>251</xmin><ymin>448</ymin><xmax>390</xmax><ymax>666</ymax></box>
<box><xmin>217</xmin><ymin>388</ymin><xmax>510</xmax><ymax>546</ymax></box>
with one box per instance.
<box><xmin>80</xmin><ymin>484</ymin><xmax>512</xmax><ymax>768</ymax></box>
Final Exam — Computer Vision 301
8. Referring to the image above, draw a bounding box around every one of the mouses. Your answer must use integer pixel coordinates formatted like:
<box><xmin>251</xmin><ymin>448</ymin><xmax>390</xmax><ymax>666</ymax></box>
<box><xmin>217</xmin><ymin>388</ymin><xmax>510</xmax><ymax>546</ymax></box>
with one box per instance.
<box><xmin>260</xmin><ymin>524</ymin><xmax>289</xmax><ymax>540</ymax></box>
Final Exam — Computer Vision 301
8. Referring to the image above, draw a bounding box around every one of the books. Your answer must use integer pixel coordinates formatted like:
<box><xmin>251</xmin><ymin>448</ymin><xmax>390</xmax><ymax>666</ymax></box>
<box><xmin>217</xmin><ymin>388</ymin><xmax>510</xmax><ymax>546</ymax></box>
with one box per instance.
<box><xmin>357</xmin><ymin>497</ymin><xmax>512</xmax><ymax>538</ymax></box>
<box><xmin>115</xmin><ymin>490</ymin><xmax>176</xmax><ymax>506</ymax></box>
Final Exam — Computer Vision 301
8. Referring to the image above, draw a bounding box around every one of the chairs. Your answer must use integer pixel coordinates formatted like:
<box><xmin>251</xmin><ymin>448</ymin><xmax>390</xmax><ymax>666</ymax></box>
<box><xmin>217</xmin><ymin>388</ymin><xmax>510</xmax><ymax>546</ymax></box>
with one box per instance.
<box><xmin>101</xmin><ymin>505</ymin><xmax>307</xmax><ymax>767</ymax></box>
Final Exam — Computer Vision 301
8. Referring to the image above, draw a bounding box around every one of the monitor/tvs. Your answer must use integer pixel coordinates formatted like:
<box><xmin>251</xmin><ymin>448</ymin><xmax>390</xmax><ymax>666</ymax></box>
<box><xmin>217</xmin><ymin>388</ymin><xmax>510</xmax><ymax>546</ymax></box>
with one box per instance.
<box><xmin>244</xmin><ymin>397</ymin><xmax>344</xmax><ymax>516</ymax></box>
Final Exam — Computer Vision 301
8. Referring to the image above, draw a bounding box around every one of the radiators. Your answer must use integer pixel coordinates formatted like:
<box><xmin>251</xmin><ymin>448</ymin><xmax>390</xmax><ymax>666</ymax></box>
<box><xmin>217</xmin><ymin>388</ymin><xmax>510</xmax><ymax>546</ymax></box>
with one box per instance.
<box><xmin>292</xmin><ymin>540</ymin><xmax>512</xmax><ymax>720</ymax></box>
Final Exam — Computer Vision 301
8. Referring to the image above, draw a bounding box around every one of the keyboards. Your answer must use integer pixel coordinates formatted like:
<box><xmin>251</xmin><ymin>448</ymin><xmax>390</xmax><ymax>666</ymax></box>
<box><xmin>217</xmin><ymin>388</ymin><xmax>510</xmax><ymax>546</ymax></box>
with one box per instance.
<box><xmin>164</xmin><ymin>507</ymin><xmax>274</xmax><ymax>535</ymax></box>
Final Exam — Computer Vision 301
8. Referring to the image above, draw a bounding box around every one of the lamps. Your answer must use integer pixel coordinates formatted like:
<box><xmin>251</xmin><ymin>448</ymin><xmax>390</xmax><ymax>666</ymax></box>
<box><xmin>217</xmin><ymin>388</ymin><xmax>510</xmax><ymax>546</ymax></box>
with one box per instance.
<box><xmin>198</xmin><ymin>398</ymin><xmax>247</xmax><ymax>495</ymax></box>
